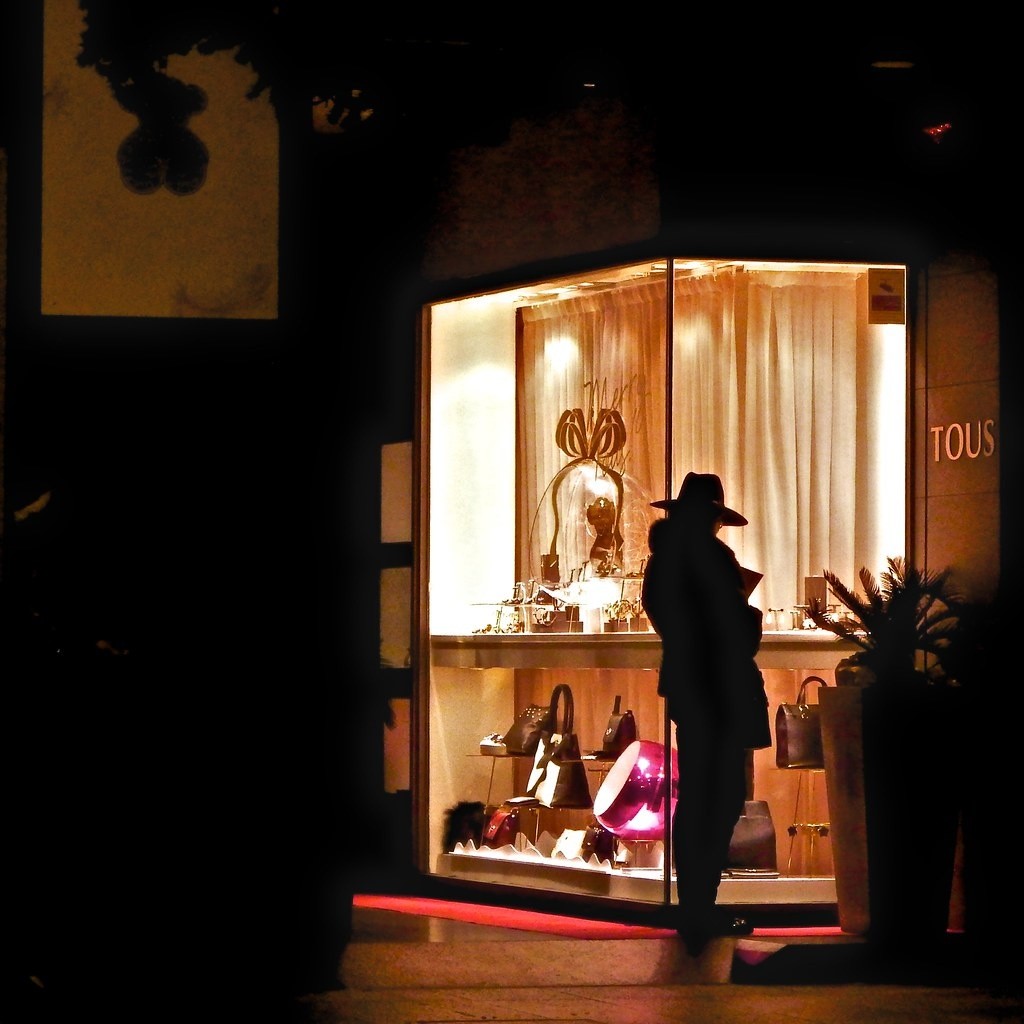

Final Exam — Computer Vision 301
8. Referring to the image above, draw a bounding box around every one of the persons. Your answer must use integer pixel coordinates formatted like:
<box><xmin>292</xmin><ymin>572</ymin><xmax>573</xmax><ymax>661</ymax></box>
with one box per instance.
<box><xmin>640</xmin><ymin>471</ymin><xmax>774</xmax><ymax>942</ymax></box>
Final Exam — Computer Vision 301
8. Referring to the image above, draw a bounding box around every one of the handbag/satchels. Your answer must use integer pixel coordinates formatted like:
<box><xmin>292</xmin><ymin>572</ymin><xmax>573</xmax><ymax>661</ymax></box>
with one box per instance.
<box><xmin>603</xmin><ymin>694</ymin><xmax>637</xmax><ymax>759</ymax></box>
<box><xmin>526</xmin><ymin>683</ymin><xmax>593</xmax><ymax>809</ymax></box>
<box><xmin>486</xmin><ymin>808</ymin><xmax>521</xmax><ymax>849</ymax></box>
<box><xmin>775</xmin><ymin>676</ymin><xmax>829</xmax><ymax>768</ymax></box>
<box><xmin>504</xmin><ymin>704</ymin><xmax>551</xmax><ymax>754</ymax></box>
<box><xmin>582</xmin><ymin>817</ymin><xmax>619</xmax><ymax>867</ymax></box>
<box><xmin>722</xmin><ymin>799</ymin><xmax>777</xmax><ymax>870</ymax></box>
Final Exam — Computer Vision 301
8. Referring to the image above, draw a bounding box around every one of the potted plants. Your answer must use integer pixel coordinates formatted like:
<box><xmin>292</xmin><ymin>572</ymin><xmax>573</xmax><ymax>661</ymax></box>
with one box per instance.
<box><xmin>808</xmin><ymin>557</ymin><xmax>988</xmax><ymax>942</ymax></box>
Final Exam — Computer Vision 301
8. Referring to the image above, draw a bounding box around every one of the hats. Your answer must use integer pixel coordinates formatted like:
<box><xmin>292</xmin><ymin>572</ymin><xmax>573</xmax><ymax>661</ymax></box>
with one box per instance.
<box><xmin>649</xmin><ymin>471</ymin><xmax>748</xmax><ymax>527</ymax></box>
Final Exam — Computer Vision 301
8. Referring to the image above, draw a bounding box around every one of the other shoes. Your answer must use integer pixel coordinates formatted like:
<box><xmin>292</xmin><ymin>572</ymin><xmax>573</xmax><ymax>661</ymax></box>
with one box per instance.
<box><xmin>714</xmin><ymin>915</ymin><xmax>754</xmax><ymax>935</ymax></box>
